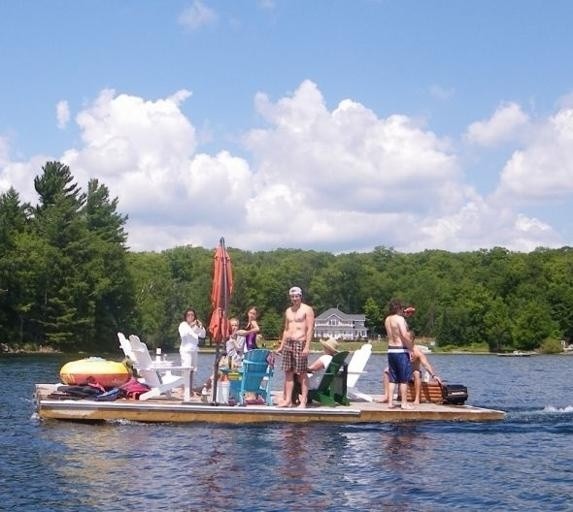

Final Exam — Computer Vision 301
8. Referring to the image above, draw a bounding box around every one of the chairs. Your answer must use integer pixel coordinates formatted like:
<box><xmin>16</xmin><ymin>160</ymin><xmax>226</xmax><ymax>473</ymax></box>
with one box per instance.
<box><xmin>117</xmin><ymin>332</ymin><xmax>374</xmax><ymax>407</ymax></box>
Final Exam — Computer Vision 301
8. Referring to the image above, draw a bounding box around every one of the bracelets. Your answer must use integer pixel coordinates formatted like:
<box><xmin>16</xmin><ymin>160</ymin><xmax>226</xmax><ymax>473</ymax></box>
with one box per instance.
<box><xmin>432</xmin><ymin>375</ymin><xmax>435</xmax><ymax>379</ymax></box>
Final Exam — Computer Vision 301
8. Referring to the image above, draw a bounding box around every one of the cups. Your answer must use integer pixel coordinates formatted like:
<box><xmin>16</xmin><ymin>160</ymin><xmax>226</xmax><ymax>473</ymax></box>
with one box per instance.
<box><xmin>163</xmin><ymin>353</ymin><xmax>168</xmax><ymax>362</ymax></box>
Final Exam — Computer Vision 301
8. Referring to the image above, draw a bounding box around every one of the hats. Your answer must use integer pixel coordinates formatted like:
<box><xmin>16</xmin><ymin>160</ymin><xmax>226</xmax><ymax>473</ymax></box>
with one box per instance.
<box><xmin>318</xmin><ymin>337</ymin><xmax>341</xmax><ymax>353</ymax></box>
<box><xmin>288</xmin><ymin>286</ymin><xmax>302</xmax><ymax>299</ymax></box>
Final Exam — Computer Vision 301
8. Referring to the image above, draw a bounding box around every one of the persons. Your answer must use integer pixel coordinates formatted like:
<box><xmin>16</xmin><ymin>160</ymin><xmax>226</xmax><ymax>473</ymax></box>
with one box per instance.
<box><xmin>383</xmin><ymin>299</ymin><xmax>415</xmax><ymax>409</ymax></box>
<box><xmin>276</xmin><ymin>333</ymin><xmax>339</xmax><ymax>403</ymax></box>
<box><xmin>273</xmin><ymin>286</ymin><xmax>309</xmax><ymax>409</ymax></box>
<box><xmin>371</xmin><ymin>328</ymin><xmax>442</xmax><ymax>404</ymax></box>
<box><xmin>179</xmin><ymin>309</ymin><xmax>207</xmax><ymax>400</ymax></box>
<box><xmin>225</xmin><ymin>316</ymin><xmax>247</xmax><ymax>369</ymax></box>
<box><xmin>243</xmin><ymin>304</ymin><xmax>262</xmax><ymax>349</ymax></box>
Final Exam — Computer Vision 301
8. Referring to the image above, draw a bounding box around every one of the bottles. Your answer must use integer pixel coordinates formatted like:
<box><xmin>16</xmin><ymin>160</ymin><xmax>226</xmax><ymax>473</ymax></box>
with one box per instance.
<box><xmin>200</xmin><ymin>383</ymin><xmax>207</xmax><ymax>401</ymax></box>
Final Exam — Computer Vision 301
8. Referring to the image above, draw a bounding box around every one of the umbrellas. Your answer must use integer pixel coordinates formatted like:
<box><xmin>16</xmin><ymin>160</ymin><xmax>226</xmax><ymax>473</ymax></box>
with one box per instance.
<box><xmin>208</xmin><ymin>234</ymin><xmax>233</xmax><ymax>404</ymax></box>
